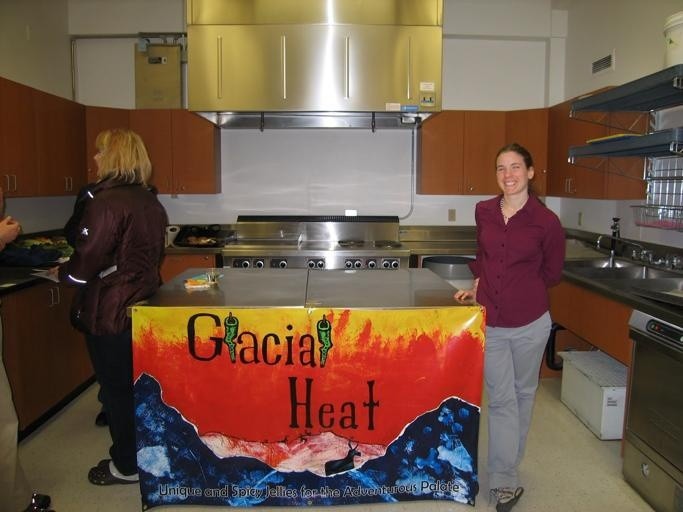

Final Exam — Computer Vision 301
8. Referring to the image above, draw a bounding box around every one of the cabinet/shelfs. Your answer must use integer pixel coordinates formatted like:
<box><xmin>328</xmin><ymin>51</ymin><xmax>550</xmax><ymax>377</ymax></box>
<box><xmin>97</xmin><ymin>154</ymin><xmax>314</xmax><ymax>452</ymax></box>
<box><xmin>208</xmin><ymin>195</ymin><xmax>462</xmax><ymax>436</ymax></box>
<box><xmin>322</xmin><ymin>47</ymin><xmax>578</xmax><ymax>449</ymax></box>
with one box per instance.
<box><xmin>130</xmin><ymin>110</ymin><xmax>220</xmax><ymax>198</ymax></box>
<box><xmin>86</xmin><ymin>107</ymin><xmax>129</xmax><ymax>191</ymax></box>
<box><xmin>548</xmin><ymin>86</ymin><xmax>648</xmax><ymax>199</ymax></box>
<box><xmin>0</xmin><ymin>77</ymin><xmax>86</xmax><ymax>199</ymax></box>
<box><xmin>2</xmin><ymin>276</ymin><xmax>98</xmax><ymax>444</ymax></box>
<box><xmin>505</xmin><ymin>108</ymin><xmax>548</xmax><ymax>195</ymax></box>
<box><xmin>416</xmin><ymin>110</ymin><xmax>506</xmax><ymax>195</ymax></box>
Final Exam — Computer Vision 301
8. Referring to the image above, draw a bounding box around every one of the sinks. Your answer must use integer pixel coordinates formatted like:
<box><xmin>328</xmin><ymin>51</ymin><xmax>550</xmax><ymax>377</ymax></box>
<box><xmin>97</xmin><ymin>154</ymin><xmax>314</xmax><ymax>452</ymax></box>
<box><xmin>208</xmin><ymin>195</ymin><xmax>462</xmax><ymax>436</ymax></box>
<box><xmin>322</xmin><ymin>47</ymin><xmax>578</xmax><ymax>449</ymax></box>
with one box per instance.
<box><xmin>578</xmin><ymin>266</ymin><xmax>682</xmax><ymax>280</ymax></box>
<box><xmin>564</xmin><ymin>256</ymin><xmax>634</xmax><ymax>269</ymax></box>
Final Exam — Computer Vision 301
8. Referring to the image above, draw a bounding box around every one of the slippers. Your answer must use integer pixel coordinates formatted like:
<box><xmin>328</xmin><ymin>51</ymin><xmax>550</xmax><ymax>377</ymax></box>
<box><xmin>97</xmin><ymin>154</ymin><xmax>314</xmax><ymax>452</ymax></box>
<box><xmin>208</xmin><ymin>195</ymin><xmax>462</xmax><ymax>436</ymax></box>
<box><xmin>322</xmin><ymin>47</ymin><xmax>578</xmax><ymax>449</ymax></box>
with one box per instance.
<box><xmin>88</xmin><ymin>459</ymin><xmax>139</xmax><ymax>485</ymax></box>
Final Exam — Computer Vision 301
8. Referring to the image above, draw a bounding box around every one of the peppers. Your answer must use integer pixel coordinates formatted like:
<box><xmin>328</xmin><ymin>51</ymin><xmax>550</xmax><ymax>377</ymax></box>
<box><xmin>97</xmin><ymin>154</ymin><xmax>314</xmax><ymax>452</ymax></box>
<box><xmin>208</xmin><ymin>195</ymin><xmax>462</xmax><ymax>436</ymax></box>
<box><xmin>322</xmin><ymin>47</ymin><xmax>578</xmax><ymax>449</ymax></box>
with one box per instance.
<box><xmin>317</xmin><ymin>315</ymin><xmax>333</xmax><ymax>368</ymax></box>
<box><xmin>224</xmin><ymin>312</ymin><xmax>240</xmax><ymax>364</ymax></box>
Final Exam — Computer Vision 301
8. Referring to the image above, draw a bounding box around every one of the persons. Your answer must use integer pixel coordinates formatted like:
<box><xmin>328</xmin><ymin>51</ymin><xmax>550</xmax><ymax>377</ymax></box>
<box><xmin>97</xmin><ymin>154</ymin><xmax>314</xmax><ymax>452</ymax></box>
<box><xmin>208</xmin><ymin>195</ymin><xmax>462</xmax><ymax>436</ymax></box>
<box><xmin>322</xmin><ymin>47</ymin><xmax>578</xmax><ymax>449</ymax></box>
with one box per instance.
<box><xmin>0</xmin><ymin>188</ymin><xmax>49</xmax><ymax>512</ymax></box>
<box><xmin>454</xmin><ymin>144</ymin><xmax>564</xmax><ymax>512</ymax></box>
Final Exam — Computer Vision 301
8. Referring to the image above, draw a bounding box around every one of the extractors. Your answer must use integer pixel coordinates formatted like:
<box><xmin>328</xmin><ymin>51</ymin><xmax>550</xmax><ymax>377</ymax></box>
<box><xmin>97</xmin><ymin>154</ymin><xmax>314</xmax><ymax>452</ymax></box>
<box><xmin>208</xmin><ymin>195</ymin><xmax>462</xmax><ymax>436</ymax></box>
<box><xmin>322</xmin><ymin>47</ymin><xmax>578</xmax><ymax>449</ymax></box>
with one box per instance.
<box><xmin>180</xmin><ymin>0</ymin><xmax>444</xmax><ymax>135</ymax></box>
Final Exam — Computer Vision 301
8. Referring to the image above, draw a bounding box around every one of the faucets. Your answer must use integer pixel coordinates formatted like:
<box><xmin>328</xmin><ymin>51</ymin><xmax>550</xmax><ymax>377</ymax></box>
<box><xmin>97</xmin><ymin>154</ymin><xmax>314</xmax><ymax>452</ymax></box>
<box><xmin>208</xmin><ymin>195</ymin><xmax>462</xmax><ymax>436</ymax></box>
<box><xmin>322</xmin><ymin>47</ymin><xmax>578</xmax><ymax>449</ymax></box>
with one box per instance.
<box><xmin>594</xmin><ymin>234</ymin><xmax>649</xmax><ymax>261</ymax></box>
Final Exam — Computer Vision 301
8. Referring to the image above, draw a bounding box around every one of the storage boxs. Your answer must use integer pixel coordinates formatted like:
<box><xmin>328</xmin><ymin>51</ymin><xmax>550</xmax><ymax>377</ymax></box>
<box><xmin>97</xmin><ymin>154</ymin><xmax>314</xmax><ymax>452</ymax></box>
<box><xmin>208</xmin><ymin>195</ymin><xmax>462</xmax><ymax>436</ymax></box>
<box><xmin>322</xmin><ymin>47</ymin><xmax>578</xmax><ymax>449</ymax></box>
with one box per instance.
<box><xmin>556</xmin><ymin>349</ymin><xmax>629</xmax><ymax>442</ymax></box>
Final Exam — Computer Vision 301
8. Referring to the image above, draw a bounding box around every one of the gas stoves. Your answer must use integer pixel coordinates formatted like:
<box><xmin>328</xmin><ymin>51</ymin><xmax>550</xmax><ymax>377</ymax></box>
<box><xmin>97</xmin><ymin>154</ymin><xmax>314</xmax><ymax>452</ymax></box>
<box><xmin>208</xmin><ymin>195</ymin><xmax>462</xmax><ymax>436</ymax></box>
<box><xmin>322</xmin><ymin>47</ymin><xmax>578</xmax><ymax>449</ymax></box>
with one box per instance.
<box><xmin>336</xmin><ymin>237</ymin><xmax>405</xmax><ymax>251</ymax></box>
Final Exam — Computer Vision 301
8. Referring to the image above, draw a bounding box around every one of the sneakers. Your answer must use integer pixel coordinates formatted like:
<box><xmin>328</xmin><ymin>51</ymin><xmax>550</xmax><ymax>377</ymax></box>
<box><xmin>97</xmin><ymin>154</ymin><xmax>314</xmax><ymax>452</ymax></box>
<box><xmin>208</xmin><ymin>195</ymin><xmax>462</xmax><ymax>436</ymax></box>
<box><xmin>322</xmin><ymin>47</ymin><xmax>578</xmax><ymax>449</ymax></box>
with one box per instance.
<box><xmin>96</xmin><ymin>410</ymin><xmax>108</xmax><ymax>427</ymax></box>
<box><xmin>487</xmin><ymin>487</ymin><xmax>524</xmax><ymax>512</ymax></box>
<box><xmin>25</xmin><ymin>494</ymin><xmax>53</xmax><ymax>512</ymax></box>
